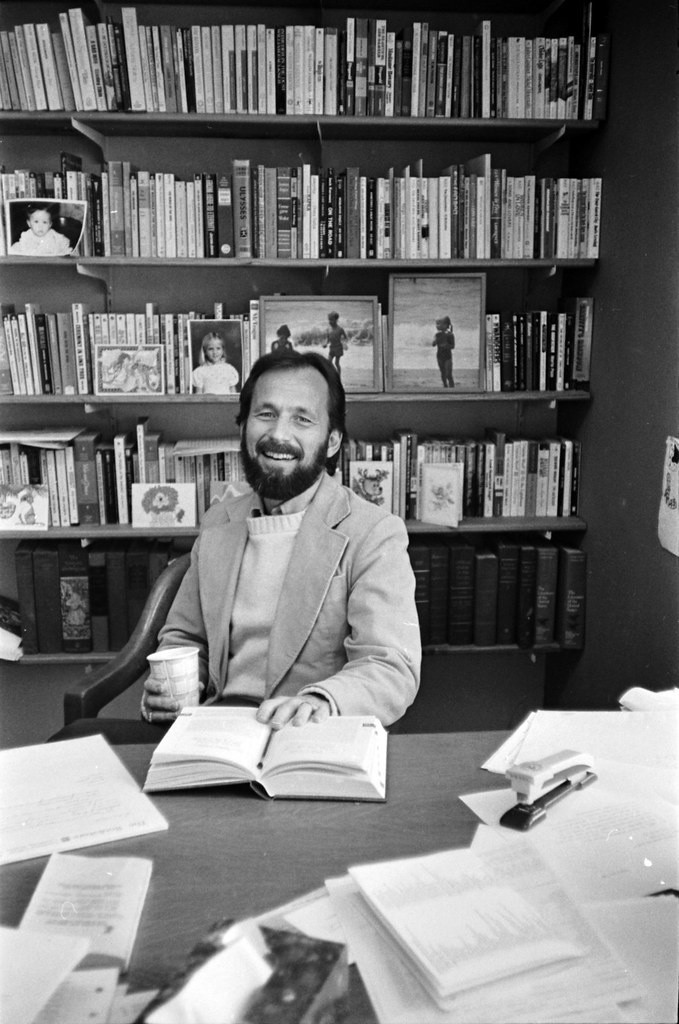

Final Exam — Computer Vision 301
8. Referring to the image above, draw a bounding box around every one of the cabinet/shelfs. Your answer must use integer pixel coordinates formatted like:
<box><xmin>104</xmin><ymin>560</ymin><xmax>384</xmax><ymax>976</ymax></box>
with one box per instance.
<box><xmin>0</xmin><ymin>0</ymin><xmax>605</xmax><ymax>748</ymax></box>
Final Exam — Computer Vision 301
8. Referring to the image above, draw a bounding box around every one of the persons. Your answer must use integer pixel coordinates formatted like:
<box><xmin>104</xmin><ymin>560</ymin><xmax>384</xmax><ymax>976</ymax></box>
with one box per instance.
<box><xmin>141</xmin><ymin>352</ymin><xmax>422</xmax><ymax>725</ymax></box>
<box><xmin>431</xmin><ymin>316</ymin><xmax>456</xmax><ymax>388</ymax></box>
<box><xmin>12</xmin><ymin>202</ymin><xmax>73</xmax><ymax>255</ymax></box>
<box><xmin>192</xmin><ymin>331</ymin><xmax>239</xmax><ymax>394</ymax></box>
<box><xmin>319</xmin><ymin>311</ymin><xmax>349</xmax><ymax>376</ymax></box>
<box><xmin>271</xmin><ymin>324</ymin><xmax>294</xmax><ymax>352</ymax></box>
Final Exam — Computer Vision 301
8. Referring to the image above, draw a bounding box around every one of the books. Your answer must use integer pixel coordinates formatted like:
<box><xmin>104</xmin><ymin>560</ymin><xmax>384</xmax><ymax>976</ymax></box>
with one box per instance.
<box><xmin>141</xmin><ymin>706</ymin><xmax>389</xmax><ymax>804</ymax></box>
<box><xmin>0</xmin><ymin>683</ymin><xmax>679</xmax><ymax>1024</ymax></box>
<box><xmin>0</xmin><ymin>6</ymin><xmax>603</xmax><ymax>651</ymax></box>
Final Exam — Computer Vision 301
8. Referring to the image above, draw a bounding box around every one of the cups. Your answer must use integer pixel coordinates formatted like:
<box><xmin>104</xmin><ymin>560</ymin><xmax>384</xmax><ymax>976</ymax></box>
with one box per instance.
<box><xmin>146</xmin><ymin>647</ymin><xmax>200</xmax><ymax>715</ymax></box>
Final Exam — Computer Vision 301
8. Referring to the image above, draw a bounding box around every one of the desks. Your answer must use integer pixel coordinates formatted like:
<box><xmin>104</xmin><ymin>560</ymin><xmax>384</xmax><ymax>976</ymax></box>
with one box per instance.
<box><xmin>0</xmin><ymin>730</ymin><xmax>516</xmax><ymax>1024</ymax></box>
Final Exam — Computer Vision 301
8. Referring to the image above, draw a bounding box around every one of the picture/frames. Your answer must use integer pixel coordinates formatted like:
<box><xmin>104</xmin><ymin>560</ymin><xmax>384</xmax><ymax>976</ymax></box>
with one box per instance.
<box><xmin>259</xmin><ymin>294</ymin><xmax>382</xmax><ymax>393</ymax></box>
<box><xmin>385</xmin><ymin>272</ymin><xmax>485</xmax><ymax>393</ymax></box>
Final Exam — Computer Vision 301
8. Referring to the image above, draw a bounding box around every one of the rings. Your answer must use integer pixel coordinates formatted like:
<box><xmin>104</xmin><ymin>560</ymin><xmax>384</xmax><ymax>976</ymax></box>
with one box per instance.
<box><xmin>300</xmin><ymin>701</ymin><xmax>313</xmax><ymax>712</ymax></box>
<box><xmin>148</xmin><ymin>712</ymin><xmax>152</xmax><ymax>722</ymax></box>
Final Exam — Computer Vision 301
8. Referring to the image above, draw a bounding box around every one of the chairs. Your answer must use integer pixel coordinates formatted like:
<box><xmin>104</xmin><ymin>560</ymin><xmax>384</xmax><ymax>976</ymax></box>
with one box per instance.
<box><xmin>63</xmin><ymin>555</ymin><xmax>194</xmax><ymax>718</ymax></box>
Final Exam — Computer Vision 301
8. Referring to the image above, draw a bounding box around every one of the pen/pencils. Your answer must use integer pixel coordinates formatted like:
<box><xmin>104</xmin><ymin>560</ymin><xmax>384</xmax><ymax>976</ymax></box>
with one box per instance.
<box><xmin>257</xmin><ymin>727</ymin><xmax>275</xmax><ymax>769</ymax></box>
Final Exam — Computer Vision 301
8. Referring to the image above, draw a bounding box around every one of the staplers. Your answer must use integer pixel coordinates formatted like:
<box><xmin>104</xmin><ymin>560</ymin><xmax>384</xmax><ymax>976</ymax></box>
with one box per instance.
<box><xmin>498</xmin><ymin>747</ymin><xmax>599</xmax><ymax>832</ymax></box>
